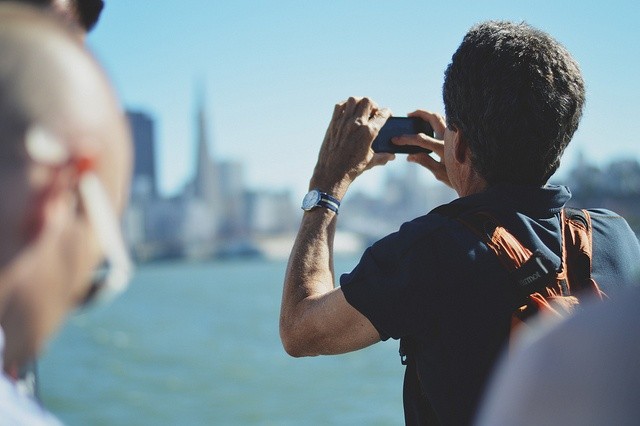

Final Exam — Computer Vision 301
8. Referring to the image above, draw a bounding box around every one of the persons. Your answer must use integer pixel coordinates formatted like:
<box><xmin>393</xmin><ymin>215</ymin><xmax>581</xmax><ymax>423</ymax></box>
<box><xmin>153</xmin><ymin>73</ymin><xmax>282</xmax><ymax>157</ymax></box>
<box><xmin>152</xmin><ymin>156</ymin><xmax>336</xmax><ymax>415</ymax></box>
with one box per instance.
<box><xmin>474</xmin><ymin>279</ymin><xmax>639</xmax><ymax>426</ymax></box>
<box><xmin>281</xmin><ymin>20</ymin><xmax>639</xmax><ymax>425</ymax></box>
<box><xmin>0</xmin><ymin>0</ymin><xmax>133</xmax><ymax>426</ymax></box>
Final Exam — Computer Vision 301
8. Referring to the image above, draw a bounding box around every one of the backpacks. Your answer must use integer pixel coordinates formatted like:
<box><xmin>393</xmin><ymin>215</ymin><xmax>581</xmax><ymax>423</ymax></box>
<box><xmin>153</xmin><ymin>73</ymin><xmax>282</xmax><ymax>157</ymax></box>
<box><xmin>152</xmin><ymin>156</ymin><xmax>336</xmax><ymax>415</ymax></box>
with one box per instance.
<box><xmin>433</xmin><ymin>199</ymin><xmax>610</xmax><ymax>357</ymax></box>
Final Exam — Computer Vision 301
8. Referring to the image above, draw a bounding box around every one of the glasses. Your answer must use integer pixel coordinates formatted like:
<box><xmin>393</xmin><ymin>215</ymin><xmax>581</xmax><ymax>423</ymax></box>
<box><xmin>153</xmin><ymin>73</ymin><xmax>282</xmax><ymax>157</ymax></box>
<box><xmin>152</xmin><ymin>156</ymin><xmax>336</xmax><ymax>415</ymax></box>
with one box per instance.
<box><xmin>20</xmin><ymin>122</ymin><xmax>132</xmax><ymax>310</ymax></box>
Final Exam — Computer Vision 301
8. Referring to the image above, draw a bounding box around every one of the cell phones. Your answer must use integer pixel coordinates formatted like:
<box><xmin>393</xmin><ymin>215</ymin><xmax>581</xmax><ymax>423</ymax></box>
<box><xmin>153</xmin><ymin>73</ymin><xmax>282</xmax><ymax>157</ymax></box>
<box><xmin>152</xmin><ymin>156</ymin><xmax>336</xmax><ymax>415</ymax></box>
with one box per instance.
<box><xmin>372</xmin><ymin>117</ymin><xmax>435</xmax><ymax>154</ymax></box>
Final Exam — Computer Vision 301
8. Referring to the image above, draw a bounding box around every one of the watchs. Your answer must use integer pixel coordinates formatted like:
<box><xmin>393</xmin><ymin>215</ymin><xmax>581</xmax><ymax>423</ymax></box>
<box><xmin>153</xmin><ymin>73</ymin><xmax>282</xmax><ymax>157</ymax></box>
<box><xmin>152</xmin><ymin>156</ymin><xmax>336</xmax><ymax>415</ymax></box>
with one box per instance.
<box><xmin>302</xmin><ymin>189</ymin><xmax>340</xmax><ymax>215</ymax></box>
